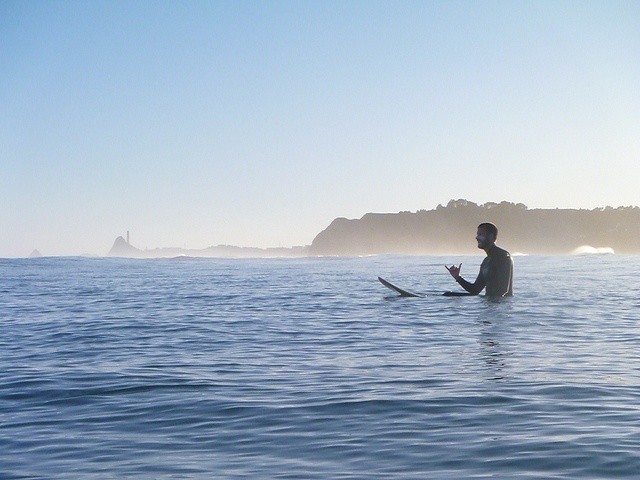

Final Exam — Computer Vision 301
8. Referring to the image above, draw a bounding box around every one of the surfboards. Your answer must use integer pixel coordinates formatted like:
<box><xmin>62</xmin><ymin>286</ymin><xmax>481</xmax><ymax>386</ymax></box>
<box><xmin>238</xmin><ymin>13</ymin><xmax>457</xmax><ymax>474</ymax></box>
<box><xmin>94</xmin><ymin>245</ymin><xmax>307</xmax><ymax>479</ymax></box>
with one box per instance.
<box><xmin>377</xmin><ymin>277</ymin><xmax>420</xmax><ymax>296</ymax></box>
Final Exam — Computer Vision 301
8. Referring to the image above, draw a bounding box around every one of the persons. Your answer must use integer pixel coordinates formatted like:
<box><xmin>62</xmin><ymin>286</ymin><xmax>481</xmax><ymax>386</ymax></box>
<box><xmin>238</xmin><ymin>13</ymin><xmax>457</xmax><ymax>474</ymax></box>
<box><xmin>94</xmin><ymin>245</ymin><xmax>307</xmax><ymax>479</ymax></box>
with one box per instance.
<box><xmin>444</xmin><ymin>223</ymin><xmax>513</xmax><ymax>297</ymax></box>
